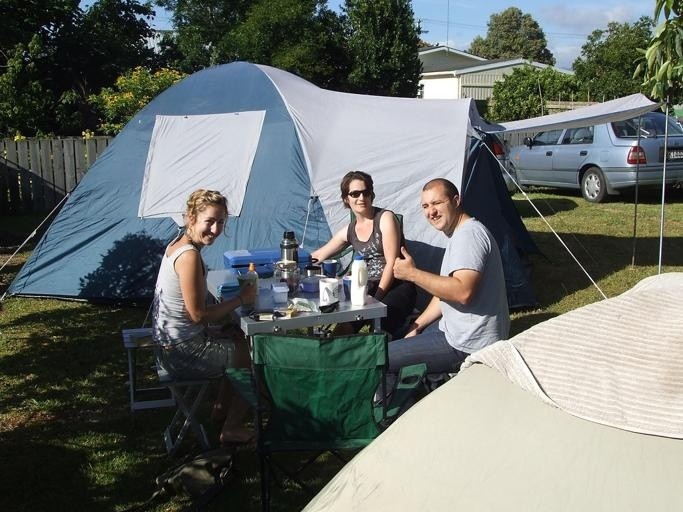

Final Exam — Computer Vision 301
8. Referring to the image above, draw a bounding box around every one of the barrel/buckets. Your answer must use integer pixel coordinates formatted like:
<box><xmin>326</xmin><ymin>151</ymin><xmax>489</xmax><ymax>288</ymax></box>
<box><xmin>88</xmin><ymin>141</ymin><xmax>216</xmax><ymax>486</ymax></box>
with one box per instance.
<box><xmin>351</xmin><ymin>256</ymin><xmax>369</xmax><ymax>305</ymax></box>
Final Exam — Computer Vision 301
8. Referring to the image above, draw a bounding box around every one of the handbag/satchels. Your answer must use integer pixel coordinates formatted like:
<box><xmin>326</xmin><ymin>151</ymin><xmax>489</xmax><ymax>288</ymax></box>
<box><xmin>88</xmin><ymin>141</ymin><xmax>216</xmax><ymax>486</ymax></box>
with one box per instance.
<box><xmin>153</xmin><ymin>435</ymin><xmax>234</xmax><ymax>498</ymax></box>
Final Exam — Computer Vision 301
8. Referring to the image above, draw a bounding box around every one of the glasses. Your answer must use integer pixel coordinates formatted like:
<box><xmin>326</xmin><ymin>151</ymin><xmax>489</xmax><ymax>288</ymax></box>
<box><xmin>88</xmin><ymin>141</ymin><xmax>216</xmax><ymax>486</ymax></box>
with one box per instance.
<box><xmin>347</xmin><ymin>189</ymin><xmax>371</xmax><ymax>198</ymax></box>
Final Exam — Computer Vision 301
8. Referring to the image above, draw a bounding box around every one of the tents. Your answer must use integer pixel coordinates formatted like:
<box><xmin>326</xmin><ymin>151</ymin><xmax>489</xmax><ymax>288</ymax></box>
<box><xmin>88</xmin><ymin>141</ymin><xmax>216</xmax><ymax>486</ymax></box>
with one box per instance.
<box><xmin>296</xmin><ymin>272</ymin><xmax>681</xmax><ymax>512</ymax></box>
<box><xmin>7</xmin><ymin>61</ymin><xmax>568</xmax><ymax>309</ymax></box>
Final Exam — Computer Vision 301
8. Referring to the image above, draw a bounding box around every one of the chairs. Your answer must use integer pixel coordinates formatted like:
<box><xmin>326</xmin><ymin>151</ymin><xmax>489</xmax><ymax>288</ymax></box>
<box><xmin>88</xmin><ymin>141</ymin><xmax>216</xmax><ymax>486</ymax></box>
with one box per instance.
<box><xmin>245</xmin><ymin>329</ymin><xmax>432</xmax><ymax>510</ymax></box>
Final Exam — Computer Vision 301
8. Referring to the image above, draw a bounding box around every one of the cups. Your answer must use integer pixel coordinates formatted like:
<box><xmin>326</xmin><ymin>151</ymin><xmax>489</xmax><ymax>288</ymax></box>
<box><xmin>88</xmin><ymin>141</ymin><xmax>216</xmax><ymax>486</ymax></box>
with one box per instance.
<box><xmin>237</xmin><ymin>274</ymin><xmax>257</xmax><ymax>305</ymax></box>
<box><xmin>319</xmin><ymin>278</ymin><xmax>339</xmax><ymax>313</ymax></box>
<box><xmin>321</xmin><ymin>259</ymin><xmax>342</xmax><ymax>279</ymax></box>
<box><xmin>343</xmin><ymin>276</ymin><xmax>351</xmax><ymax>300</ymax></box>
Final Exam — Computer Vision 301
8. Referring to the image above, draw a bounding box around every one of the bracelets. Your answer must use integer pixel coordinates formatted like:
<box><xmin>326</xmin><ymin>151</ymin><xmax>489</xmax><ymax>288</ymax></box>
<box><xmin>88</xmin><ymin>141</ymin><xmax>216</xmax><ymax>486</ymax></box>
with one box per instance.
<box><xmin>235</xmin><ymin>294</ymin><xmax>244</xmax><ymax>306</ymax></box>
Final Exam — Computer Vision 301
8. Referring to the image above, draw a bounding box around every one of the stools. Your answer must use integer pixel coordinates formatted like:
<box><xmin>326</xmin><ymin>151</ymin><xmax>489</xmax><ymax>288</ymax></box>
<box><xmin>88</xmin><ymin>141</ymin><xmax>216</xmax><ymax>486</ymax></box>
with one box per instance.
<box><xmin>151</xmin><ymin>365</ymin><xmax>213</xmax><ymax>465</ymax></box>
<box><xmin>121</xmin><ymin>327</ymin><xmax>176</xmax><ymax>414</ymax></box>
<box><xmin>383</xmin><ymin>308</ymin><xmax>423</xmax><ymax>340</ymax></box>
<box><xmin>423</xmin><ymin>372</ymin><xmax>457</xmax><ymax>393</ymax></box>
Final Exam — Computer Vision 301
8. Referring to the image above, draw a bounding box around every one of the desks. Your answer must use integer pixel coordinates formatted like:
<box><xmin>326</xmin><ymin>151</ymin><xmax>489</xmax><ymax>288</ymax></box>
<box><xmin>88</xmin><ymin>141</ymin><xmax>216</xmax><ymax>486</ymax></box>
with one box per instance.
<box><xmin>204</xmin><ymin>268</ymin><xmax>388</xmax><ymax>511</ymax></box>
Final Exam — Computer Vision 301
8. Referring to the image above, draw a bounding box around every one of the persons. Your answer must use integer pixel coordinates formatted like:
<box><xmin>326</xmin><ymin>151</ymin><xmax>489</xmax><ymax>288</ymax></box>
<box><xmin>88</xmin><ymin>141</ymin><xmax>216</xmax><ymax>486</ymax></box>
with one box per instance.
<box><xmin>375</xmin><ymin>176</ymin><xmax>510</xmax><ymax>409</ymax></box>
<box><xmin>152</xmin><ymin>186</ymin><xmax>258</xmax><ymax>448</ymax></box>
<box><xmin>309</xmin><ymin>170</ymin><xmax>417</xmax><ymax>337</ymax></box>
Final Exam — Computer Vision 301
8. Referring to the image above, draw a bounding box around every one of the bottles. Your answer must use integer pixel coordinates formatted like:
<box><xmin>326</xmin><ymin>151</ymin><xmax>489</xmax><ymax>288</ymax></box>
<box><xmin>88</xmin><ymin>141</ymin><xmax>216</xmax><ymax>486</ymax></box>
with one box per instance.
<box><xmin>279</xmin><ymin>231</ymin><xmax>299</xmax><ymax>262</ymax></box>
<box><xmin>246</xmin><ymin>261</ymin><xmax>259</xmax><ymax>296</ymax></box>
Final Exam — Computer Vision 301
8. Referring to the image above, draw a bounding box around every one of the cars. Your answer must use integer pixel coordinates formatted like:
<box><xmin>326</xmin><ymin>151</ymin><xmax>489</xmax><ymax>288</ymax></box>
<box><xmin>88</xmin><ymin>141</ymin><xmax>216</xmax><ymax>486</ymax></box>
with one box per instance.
<box><xmin>507</xmin><ymin>111</ymin><xmax>683</xmax><ymax>202</ymax></box>
<box><xmin>487</xmin><ymin>122</ymin><xmax>519</xmax><ymax>198</ymax></box>
